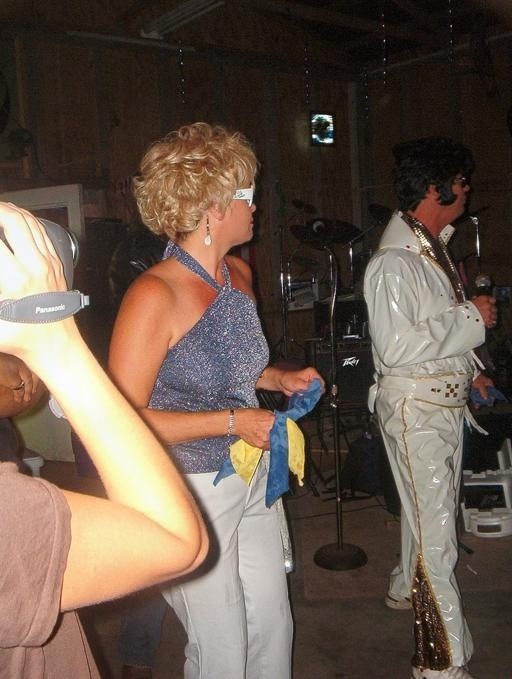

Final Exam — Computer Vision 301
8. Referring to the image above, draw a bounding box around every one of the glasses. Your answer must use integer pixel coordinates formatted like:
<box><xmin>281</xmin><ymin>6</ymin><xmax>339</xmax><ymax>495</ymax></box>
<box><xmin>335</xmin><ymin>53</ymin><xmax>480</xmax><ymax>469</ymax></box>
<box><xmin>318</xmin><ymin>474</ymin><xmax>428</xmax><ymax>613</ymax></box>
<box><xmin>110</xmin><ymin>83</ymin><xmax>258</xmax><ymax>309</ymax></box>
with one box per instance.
<box><xmin>232</xmin><ymin>180</ymin><xmax>256</xmax><ymax>207</ymax></box>
<box><xmin>455</xmin><ymin>176</ymin><xmax>472</xmax><ymax>187</ymax></box>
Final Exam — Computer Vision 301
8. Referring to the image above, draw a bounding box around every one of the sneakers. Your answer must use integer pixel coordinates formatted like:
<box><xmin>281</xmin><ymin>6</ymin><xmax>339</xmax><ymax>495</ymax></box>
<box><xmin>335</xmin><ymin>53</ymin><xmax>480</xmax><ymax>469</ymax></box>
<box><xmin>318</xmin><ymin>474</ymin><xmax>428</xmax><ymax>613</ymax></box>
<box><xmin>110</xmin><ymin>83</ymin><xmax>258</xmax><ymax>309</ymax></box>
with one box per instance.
<box><xmin>385</xmin><ymin>584</ymin><xmax>414</xmax><ymax>609</ymax></box>
<box><xmin>412</xmin><ymin>659</ymin><xmax>472</xmax><ymax>679</ymax></box>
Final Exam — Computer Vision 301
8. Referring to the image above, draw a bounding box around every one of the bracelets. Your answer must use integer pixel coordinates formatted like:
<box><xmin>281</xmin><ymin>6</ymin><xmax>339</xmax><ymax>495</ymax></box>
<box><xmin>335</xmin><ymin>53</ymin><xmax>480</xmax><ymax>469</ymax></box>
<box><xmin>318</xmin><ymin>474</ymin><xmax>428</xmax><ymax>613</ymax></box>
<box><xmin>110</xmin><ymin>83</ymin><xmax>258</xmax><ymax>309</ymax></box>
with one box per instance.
<box><xmin>227</xmin><ymin>408</ymin><xmax>235</xmax><ymax>436</ymax></box>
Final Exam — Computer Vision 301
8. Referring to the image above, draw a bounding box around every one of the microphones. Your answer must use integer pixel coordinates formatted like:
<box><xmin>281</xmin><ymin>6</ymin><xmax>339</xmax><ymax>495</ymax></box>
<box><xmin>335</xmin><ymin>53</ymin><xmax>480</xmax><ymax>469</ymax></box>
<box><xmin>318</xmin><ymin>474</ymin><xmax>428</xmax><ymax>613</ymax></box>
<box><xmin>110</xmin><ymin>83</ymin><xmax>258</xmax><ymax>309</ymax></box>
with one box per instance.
<box><xmin>474</xmin><ymin>272</ymin><xmax>495</xmax><ymax>301</ymax></box>
<box><xmin>310</xmin><ymin>217</ymin><xmax>327</xmax><ymax>246</ymax></box>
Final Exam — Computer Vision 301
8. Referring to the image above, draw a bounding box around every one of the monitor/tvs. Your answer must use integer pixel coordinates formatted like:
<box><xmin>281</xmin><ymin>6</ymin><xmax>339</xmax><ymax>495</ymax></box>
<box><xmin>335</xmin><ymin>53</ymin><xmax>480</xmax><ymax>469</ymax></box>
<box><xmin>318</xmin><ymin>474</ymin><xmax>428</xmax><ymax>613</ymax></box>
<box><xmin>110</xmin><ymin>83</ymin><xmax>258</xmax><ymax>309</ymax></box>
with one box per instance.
<box><xmin>307</xmin><ymin>111</ymin><xmax>335</xmax><ymax>144</ymax></box>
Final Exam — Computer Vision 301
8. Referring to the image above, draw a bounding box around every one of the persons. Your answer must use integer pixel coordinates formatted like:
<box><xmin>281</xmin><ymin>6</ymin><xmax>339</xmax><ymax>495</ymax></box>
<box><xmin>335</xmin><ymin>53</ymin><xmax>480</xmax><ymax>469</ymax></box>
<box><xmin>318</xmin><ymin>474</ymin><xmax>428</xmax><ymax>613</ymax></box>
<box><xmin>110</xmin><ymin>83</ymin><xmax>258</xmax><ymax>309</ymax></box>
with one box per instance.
<box><xmin>0</xmin><ymin>200</ymin><xmax>210</xmax><ymax>679</ymax></box>
<box><xmin>108</xmin><ymin>120</ymin><xmax>326</xmax><ymax>679</ymax></box>
<box><xmin>360</xmin><ymin>133</ymin><xmax>499</xmax><ymax>679</ymax></box>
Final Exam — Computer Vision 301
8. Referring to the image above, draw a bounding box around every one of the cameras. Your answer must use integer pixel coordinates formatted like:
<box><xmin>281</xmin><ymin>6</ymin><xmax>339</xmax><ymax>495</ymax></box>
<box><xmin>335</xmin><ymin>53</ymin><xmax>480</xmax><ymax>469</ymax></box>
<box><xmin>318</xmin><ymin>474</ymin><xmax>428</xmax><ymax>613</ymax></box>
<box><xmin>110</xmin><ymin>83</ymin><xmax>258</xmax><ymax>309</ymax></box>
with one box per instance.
<box><xmin>0</xmin><ymin>213</ymin><xmax>92</xmax><ymax>327</ymax></box>
